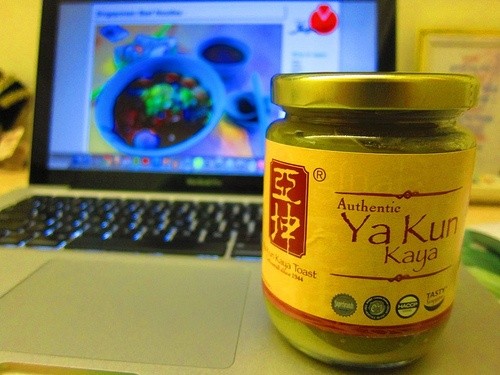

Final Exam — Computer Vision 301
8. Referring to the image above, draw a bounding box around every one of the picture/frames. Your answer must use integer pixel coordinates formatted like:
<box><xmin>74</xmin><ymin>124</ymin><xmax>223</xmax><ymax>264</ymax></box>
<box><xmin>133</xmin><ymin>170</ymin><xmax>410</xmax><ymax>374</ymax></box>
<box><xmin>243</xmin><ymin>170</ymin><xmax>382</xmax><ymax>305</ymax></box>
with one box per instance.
<box><xmin>416</xmin><ymin>28</ymin><xmax>500</xmax><ymax>202</ymax></box>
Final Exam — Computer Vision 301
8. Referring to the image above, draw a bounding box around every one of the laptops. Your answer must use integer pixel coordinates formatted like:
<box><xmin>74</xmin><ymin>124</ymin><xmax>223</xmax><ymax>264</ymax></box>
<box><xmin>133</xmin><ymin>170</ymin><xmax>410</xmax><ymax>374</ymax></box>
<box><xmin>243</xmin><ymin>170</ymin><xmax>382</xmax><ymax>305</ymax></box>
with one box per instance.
<box><xmin>0</xmin><ymin>0</ymin><xmax>500</xmax><ymax>375</ymax></box>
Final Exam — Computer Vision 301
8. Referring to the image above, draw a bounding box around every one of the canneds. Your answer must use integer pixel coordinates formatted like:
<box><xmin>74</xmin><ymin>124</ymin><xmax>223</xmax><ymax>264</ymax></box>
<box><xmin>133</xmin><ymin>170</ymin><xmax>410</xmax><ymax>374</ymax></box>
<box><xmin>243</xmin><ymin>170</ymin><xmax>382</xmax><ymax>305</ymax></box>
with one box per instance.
<box><xmin>260</xmin><ymin>73</ymin><xmax>481</xmax><ymax>368</ymax></box>
